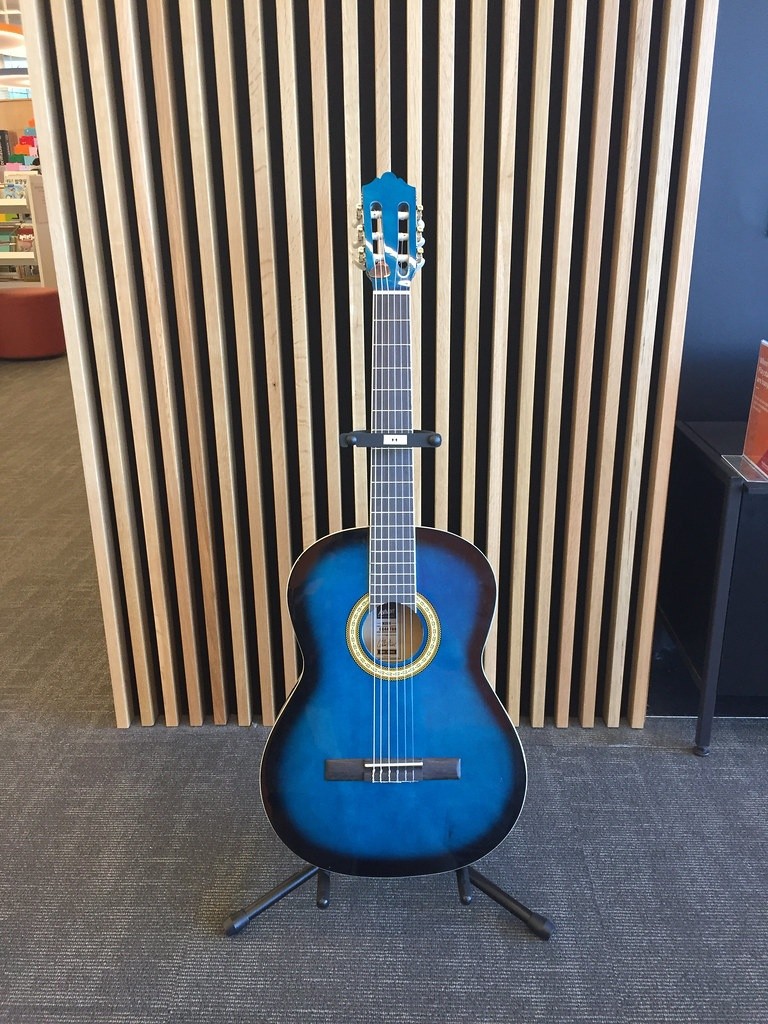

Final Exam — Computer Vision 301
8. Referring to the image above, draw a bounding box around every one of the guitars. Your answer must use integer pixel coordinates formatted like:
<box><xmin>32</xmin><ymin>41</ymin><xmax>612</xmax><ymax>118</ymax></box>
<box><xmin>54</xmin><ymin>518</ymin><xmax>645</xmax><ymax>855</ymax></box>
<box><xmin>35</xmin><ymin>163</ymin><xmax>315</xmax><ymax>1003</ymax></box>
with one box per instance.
<box><xmin>255</xmin><ymin>169</ymin><xmax>529</xmax><ymax>876</ymax></box>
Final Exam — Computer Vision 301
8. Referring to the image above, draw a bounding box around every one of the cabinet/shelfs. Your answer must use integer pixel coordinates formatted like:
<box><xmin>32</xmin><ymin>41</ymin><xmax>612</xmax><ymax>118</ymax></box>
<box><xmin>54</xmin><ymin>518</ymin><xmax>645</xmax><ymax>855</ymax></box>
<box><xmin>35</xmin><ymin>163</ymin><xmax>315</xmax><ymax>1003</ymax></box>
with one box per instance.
<box><xmin>0</xmin><ymin>175</ymin><xmax>57</xmax><ymax>288</ymax></box>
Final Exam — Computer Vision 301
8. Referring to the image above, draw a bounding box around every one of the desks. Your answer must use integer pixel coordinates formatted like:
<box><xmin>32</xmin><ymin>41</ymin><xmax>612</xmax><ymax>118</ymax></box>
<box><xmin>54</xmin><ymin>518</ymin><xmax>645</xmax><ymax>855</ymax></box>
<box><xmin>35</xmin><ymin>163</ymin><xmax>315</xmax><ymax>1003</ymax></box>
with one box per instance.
<box><xmin>657</xmin><ymin>421</ymin><xmax>768</xmax><ymax>756</ymax></box>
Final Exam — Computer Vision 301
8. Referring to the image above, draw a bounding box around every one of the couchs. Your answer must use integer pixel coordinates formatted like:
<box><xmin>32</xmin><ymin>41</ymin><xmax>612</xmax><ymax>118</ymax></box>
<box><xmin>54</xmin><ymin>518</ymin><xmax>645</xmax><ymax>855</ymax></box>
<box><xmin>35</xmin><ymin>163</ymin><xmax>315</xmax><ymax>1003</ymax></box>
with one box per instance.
<box><xmin>0</xmin><ymin>288</ymin><xmax>66</xmax><ymax>360</ymax></box>
